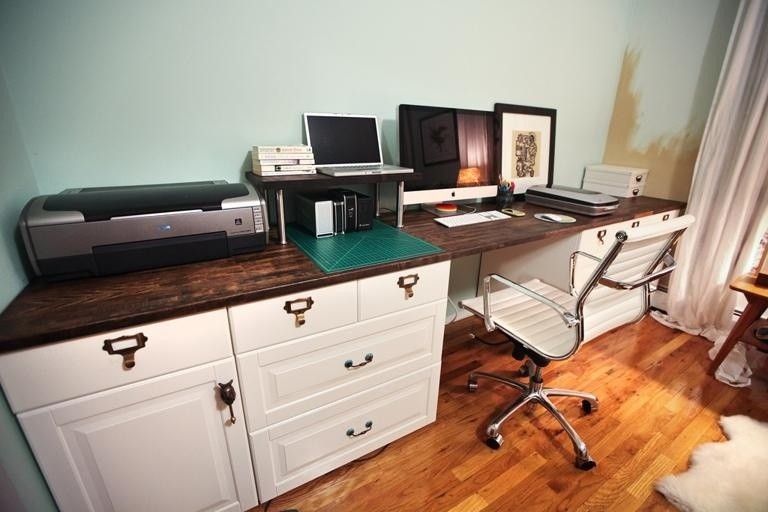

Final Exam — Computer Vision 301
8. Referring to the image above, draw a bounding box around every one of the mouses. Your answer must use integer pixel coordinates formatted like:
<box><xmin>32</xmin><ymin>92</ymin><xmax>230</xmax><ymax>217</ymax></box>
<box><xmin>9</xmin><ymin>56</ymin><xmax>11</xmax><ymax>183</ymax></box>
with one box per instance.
<box><xmin>541</xmin><ymin>212</ymin><xmax>560</xmax><ymax>223</ymax></box>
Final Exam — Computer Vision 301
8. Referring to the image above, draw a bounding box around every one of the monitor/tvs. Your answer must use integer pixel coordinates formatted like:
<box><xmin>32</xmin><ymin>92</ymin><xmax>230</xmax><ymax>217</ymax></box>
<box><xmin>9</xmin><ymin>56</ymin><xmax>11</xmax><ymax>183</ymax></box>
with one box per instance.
<box><xmin>397</xmin><ymin>102</ymin><xmax>499</xmax><ymax>221</ymax></box>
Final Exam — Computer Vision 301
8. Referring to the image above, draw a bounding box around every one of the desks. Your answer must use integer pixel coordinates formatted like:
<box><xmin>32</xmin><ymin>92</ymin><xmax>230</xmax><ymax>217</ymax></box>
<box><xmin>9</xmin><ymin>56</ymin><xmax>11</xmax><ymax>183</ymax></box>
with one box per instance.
<box><xmin>0</xmin><ymin>189</ymin><xmax>690</xmax><ymax>509</ymax></box>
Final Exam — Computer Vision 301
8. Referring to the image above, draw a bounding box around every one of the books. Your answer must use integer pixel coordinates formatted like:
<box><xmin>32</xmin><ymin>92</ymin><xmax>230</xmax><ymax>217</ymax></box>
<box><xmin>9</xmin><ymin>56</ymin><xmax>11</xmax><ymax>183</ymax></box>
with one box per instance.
<box><xmin>251</xmin><ymin>144</ymin><xmax>317</xmax><ymax>177</ymax></box>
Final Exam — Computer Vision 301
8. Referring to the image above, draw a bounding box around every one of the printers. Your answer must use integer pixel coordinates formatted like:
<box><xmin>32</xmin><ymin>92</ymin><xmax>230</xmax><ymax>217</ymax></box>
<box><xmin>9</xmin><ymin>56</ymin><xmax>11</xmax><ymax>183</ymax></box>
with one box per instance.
<box><xmin>12</xmin><ymin>178</ymin><xmax>272</xmax><ymax>291</ymax></box>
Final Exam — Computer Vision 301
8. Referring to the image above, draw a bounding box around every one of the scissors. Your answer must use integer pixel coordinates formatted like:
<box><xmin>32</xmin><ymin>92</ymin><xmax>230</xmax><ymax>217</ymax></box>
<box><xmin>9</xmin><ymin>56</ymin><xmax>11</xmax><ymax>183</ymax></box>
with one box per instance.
<box><xmin>500</xmin><ymin>178</ymin><xmax>511</xmax><ymax>190</ymax></box>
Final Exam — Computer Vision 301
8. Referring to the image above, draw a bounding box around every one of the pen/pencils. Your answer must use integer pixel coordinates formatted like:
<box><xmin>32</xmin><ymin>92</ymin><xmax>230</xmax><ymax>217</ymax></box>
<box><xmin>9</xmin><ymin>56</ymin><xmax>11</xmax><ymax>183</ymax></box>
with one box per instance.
<box><xmin>497</xmin><ymin>174</ymin><xmax>515</xmax><ymax>209</ymax></box>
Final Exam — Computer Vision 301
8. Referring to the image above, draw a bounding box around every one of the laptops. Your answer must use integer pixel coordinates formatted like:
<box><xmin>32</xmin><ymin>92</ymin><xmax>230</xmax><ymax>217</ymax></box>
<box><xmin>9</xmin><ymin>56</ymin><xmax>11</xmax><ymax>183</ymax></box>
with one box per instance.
<box><xmin>303</xmin><ymin>112</ymin><xmax>419</xmax><ymax>182</ymax></box>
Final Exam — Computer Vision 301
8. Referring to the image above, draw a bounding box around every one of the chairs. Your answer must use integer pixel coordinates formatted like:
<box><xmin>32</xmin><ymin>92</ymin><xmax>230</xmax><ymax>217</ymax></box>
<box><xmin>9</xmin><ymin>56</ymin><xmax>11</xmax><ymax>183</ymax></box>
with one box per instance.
<box><xmin>458</xmin><ymin>214</ymin><xmax>696</xmax><ymax>469</ymax></box>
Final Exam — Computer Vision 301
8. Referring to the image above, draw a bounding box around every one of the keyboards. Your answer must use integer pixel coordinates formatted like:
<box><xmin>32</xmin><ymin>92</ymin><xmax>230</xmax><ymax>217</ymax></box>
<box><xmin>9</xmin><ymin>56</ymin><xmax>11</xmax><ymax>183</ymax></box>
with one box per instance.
<box><xmin>431</xmin><ymin>209</ymin><xmax>512</xmax><ymax>229</ymax></box>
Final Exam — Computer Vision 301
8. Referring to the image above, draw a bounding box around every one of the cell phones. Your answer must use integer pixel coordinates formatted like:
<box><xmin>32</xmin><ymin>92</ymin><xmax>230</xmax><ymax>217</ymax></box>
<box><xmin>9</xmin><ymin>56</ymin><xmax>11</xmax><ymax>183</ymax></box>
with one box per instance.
<box><xmin>501</xmin><ymin>208</ymin><xmax>526</xmax><ymax>217</ymax></box>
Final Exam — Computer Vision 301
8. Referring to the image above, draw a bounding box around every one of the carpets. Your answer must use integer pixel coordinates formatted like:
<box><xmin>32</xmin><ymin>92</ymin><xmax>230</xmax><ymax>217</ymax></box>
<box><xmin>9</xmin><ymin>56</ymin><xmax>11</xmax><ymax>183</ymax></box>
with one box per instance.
<box><xmin>652</xmin><ymin>415</ymin><xmax>768</xmax><ymax>511</ymax></box>
